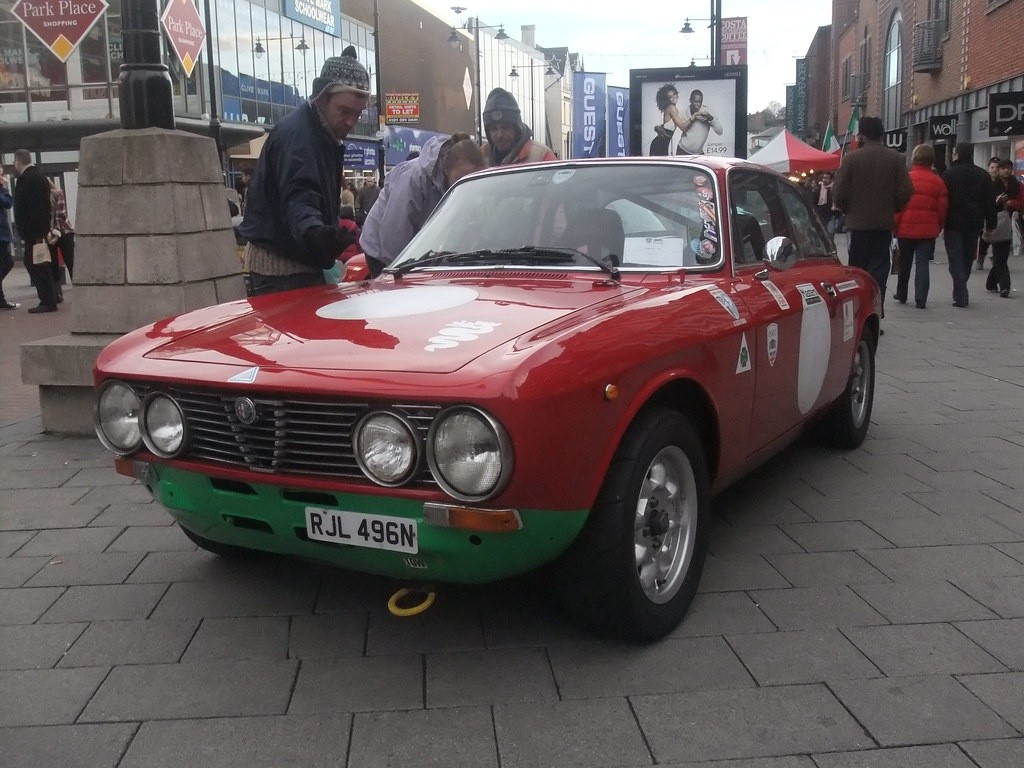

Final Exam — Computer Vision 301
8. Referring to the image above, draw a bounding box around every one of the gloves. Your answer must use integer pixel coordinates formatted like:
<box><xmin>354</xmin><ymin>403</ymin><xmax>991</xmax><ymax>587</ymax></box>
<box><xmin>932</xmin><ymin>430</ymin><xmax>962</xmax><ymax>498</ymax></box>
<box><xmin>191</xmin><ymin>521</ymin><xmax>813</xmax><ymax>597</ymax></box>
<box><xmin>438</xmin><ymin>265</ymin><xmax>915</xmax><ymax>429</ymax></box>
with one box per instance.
<box><xmin>302</xmin><ymin>226</ymin><xmax>338</xmax><ymax>269</ymax></box>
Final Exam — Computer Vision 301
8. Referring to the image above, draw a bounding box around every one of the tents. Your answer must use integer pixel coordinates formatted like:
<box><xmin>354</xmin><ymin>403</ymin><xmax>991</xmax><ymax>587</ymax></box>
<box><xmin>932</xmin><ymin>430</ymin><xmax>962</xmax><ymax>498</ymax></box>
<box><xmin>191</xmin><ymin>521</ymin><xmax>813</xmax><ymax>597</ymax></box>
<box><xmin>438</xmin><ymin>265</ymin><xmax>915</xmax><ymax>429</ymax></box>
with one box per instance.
<box><xmin>745</xmin><ymin>126</ymin><xmax>840</xmax><ymax>178</ymax></box>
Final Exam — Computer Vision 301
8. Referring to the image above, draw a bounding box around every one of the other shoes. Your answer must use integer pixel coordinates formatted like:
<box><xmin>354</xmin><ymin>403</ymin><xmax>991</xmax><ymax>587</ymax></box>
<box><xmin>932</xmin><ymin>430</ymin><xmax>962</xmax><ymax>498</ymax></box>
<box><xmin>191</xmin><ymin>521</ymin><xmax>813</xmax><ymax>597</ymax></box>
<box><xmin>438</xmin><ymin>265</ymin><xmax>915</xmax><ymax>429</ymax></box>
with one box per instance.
<box><xmin>976</xmin><ymin>263</ymin><xmax>983</xmax><ymax>270</ymax></box>
<box><xmin>915</xmin><ymin>300</ymin><xmax>926</xmax><ymax>308</ymax></box>
<box><xmin>28</xmin><ymin>303</ymin><xmax>57</xmax><ymax>314</ymax></box>
<box><xmin>893</xmin><ymin>293</ymin><xmax>908</xmax><ymax>304</ymax></box>
<box><xmin>952</xmin><ymin>296</ymin><xmax>967</xmax><ymax>307</ymax></box>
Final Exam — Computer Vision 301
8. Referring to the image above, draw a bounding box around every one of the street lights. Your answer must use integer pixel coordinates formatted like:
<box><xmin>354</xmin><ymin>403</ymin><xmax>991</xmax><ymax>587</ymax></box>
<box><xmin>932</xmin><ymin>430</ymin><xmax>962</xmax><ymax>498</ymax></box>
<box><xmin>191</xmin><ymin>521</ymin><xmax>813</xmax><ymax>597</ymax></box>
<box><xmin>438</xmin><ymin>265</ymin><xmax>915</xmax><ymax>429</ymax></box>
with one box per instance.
<box><xmin>510</xmin><ymin>59</ymin><xmax>557</xmax><ymax>147</ymax></box>
<box><xmin>681</xmin><ymin>13</ymin><xmax>725</xmax><ymax>65</ymax></box>
<box><xmin>447</xmin><ymin>21</ymin><xmax>507</xmax><ymax>147</ymax></box>
<box><xmin>251</xmin><ymin>34</ymin><xmax>311</xmax><ymax>125</ymax></box>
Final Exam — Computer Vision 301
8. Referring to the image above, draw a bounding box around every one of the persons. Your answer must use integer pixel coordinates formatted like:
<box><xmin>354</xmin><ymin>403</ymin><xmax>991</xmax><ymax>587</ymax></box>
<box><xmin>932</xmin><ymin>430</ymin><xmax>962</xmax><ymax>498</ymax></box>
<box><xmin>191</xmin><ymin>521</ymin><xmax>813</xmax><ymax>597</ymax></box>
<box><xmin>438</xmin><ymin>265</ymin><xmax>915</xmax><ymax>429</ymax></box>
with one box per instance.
<box><xmin>647</xmin><ymin>84</ymin><xmax>723</xmax><ymax>157</ymax></box>
<box><xmin>216</xmin><ymin>150</ymin><xmax>426</xmax><ymax>263</ymax></box>
<box><xmin>245</xmin><ymin>55</ymin><xmax>369</xmax><ymax>296</ymax></box>
<box><xmin>364</xmin><ymin>133</ymin><xmax>484</xmax><ymax>278</ymax></box>
<box><xmin>0</xmin><ymin>149</ymin><xmax>77</xmax><ymax>314</ymax></box>
<box><xmin>480</xmin><ymin>90</ymin><xmax>560</xmax><ymax>164</ymax></box>
<box><xmin>792</xmin><ymin>116</ymin><xmax>1024</xmax><ymax>335</ymax></box>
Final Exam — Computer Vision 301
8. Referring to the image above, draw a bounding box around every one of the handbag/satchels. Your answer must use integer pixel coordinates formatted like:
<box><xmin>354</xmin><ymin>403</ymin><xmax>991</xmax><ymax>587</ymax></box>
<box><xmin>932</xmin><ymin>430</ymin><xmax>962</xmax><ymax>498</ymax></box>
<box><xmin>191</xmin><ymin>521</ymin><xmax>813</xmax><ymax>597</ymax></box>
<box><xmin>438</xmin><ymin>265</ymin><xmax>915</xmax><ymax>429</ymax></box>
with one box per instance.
<box><xmin>983</xmin><ymin>195</ymin><xmax>1013</xmax><ymax>242</ymax></box>
<box><xmin>890</xmin><ymin>249</ymin><xmax>904</xmax><ymax>275</ymax></box>
<box><xmin>32</xmin><ymin>239</ymin><xmax>52</xmax><ymax>264</ymax></box>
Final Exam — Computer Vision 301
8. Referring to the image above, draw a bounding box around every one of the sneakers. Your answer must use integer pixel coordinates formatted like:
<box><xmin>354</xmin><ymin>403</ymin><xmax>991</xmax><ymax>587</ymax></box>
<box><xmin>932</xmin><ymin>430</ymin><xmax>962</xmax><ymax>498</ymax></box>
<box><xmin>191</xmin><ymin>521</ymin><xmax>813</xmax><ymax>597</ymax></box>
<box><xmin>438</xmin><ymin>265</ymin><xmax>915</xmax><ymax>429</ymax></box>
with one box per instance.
<box><xmin>0</xmin><ymin>302</ymin><xmax>21</xmax><ymax>310</ymax></box>
<box><xmin>990</xmin><ymin>289</ymin><xmax>1009</xmax><ymax>297</ymax></box>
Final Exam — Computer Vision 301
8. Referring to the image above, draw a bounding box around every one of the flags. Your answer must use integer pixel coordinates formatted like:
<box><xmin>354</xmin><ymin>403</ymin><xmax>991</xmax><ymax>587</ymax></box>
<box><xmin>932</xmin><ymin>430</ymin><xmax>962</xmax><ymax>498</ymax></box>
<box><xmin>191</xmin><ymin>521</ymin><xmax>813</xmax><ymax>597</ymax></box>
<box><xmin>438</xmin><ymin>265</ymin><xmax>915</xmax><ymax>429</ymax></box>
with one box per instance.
<box><xmin>824</xmin><ymin>124</ymin><xmax>842</xmax><ymax>158</ymax></box>
<box><xmin>847</xmin><ymin>102</ymin><xmax>862</xmax><ymax>150</ymax></box>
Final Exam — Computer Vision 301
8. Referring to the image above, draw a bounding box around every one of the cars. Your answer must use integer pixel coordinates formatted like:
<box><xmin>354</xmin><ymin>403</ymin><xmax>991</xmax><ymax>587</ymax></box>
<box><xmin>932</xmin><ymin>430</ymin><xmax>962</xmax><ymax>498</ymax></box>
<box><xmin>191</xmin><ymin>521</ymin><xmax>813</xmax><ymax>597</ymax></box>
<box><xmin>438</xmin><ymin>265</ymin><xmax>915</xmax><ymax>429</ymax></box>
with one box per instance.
<box><xmin>92</xmin><ymin>152</ymin><xmax>888</xmax><ymax>636</ymax></box>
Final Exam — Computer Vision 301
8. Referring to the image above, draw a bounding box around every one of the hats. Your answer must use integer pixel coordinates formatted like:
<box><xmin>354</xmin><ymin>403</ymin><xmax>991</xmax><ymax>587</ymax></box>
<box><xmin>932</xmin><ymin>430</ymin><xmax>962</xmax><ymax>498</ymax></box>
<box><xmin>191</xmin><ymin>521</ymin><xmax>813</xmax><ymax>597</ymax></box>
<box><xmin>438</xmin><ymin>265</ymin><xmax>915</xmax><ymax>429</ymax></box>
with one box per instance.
<box><xmin>483</xmin><ymin>87</ymin><xmax>522</xmax><ymax>150</ymax></box>
<box><xmin>957</xmin><ymin>142</ymin><xmax>973</xmax><ymax>160</ymax></box>
<box><xmin>307</xmin><ymin>55</ymin><xmax>371</xmax><ymax>108</ymax></box>
